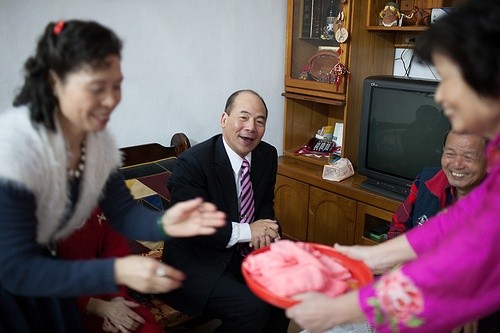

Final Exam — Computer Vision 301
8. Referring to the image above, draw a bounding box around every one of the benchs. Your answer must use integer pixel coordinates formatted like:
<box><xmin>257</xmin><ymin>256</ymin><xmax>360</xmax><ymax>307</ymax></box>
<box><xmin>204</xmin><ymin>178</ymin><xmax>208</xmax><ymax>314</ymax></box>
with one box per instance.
<box><xmin>118</xmin><ymin>132</ymin><xmax>220</xmax><ymax>333</ymax></box>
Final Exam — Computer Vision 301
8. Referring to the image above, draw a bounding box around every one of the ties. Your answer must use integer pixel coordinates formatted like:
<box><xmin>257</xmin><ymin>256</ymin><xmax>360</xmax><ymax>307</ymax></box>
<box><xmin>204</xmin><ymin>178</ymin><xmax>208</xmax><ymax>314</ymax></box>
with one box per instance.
<box><xmin>239</xmin><ymin>159</ymin><xmax>254</xmax><ymax>258</ymax></box>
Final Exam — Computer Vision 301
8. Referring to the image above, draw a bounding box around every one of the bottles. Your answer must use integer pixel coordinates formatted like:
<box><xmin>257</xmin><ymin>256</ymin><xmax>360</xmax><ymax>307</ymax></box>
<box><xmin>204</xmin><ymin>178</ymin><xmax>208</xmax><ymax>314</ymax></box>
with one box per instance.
<box><xmin>326</xmin><ymin>0</ymin><xmax>338</xmax><ymax>39</ymax></box>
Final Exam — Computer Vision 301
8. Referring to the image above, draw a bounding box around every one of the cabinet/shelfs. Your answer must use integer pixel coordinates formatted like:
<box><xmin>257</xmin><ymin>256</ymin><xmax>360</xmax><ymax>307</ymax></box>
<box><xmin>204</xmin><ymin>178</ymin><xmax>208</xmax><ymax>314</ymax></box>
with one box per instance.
<box><xmin>273</xmin><ymin>0</ymin><xmax>445</xmax><ymax>246</ymax></box>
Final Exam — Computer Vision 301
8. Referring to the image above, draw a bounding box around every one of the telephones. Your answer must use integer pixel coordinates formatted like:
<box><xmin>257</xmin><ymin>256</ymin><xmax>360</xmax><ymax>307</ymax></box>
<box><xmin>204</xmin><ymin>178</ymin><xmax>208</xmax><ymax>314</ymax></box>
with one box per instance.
<box><xmin>306</xmin><ymin>138</ymin><xmax>336</xmax><ymax>155</ymax></box>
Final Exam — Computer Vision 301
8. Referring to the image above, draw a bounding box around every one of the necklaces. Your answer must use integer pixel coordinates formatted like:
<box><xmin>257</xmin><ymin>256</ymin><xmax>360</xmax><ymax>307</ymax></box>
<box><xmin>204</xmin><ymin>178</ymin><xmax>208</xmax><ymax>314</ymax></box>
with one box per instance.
<box><xmin>66</xmin><ymin>142</ymin><xmax>85</xmax><ymax>179</ymax></box>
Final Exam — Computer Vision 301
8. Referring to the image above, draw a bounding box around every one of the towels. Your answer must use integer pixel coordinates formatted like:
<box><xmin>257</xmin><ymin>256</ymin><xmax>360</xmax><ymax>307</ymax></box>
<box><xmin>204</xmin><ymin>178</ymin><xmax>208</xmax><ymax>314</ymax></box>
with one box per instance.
<box><xmin>240</xmin><ymin>238</ymin><xmax>351</xmax><ymax>301</ymax></box>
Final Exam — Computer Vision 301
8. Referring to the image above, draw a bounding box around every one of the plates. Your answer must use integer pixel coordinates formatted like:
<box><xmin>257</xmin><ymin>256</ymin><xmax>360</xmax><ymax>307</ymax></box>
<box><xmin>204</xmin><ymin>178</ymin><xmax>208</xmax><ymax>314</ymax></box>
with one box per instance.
<box><xmin>241</xmin><ymin>241</ymin><xmax>375</xmax><ymax>309</ymax></box>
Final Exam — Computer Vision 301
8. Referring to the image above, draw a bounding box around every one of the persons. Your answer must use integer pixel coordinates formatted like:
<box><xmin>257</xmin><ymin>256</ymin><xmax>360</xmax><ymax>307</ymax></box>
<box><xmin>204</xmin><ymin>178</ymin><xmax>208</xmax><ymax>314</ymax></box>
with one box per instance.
<box><xmin>57</xmin><ymin>203</ymin><xmax>163</xmax><ymax>333</ymax></box>
<box><xmin>284</xmin><ymin>0</ymin><xmax>500</xmax><ymax>333</ymax></box>
<box><xmin>0</xmin><ymin>19</ymin><xmax>227</xmax><ymax>333</ymax></box>
<box><xmin>386</xmin><ymin>128</ymin><xmax>500</xmax><ymax>333</ymax></box>
<box><xmin>161</xmin><ymin>90</ymin><xmax>290</xmax><ymax>333</ymax></box>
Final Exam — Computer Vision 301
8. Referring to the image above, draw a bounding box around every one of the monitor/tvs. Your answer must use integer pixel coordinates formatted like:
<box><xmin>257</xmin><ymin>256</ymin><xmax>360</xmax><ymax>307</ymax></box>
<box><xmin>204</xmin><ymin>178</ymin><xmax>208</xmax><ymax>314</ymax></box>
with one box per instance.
<box><xmin>357</xmin><ymin>74</ymin><xmax>450</xmax><ymax>201</ymax></box>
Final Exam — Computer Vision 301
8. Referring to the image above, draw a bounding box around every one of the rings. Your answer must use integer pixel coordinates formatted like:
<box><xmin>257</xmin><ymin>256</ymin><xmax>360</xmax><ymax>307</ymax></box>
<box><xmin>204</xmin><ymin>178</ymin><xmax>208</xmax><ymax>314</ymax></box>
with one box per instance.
<box><xmin>128</xmin><ymin>311</ymin><xmax>136</xmax><ymax>318</ymax></box>
<box><xmin>157</xmin><ymin>264</ymin><xmax>167</xmax><ymax>277</ymax></box>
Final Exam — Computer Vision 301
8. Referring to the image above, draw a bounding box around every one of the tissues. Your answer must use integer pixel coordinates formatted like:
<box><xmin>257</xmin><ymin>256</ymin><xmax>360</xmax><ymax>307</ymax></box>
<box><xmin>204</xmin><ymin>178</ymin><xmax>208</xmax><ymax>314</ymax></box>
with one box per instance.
<box><xmin>322</xmin><ymin>157</ymin><xmax>355</xmax><ymax>182</ymax></box>
